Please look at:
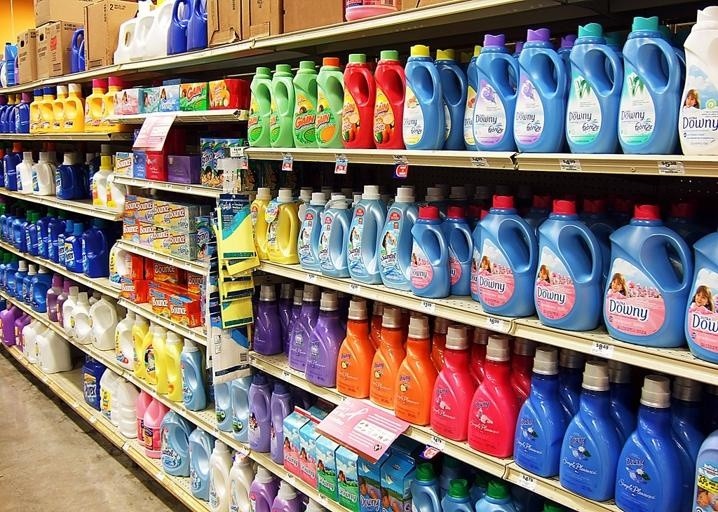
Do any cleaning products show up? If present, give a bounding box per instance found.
[1,1,718,512]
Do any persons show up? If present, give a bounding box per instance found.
[122,89,187,105]
[203,166,212,181]
[478,255,492,274]
[682,89,701,110]
[605,272,628,297]
[688,285,715,312]
[536,265,550,284]
[267,221,397,258]
[282,436,398,512]
[217,170,224,181]
[411,253,417,265]
